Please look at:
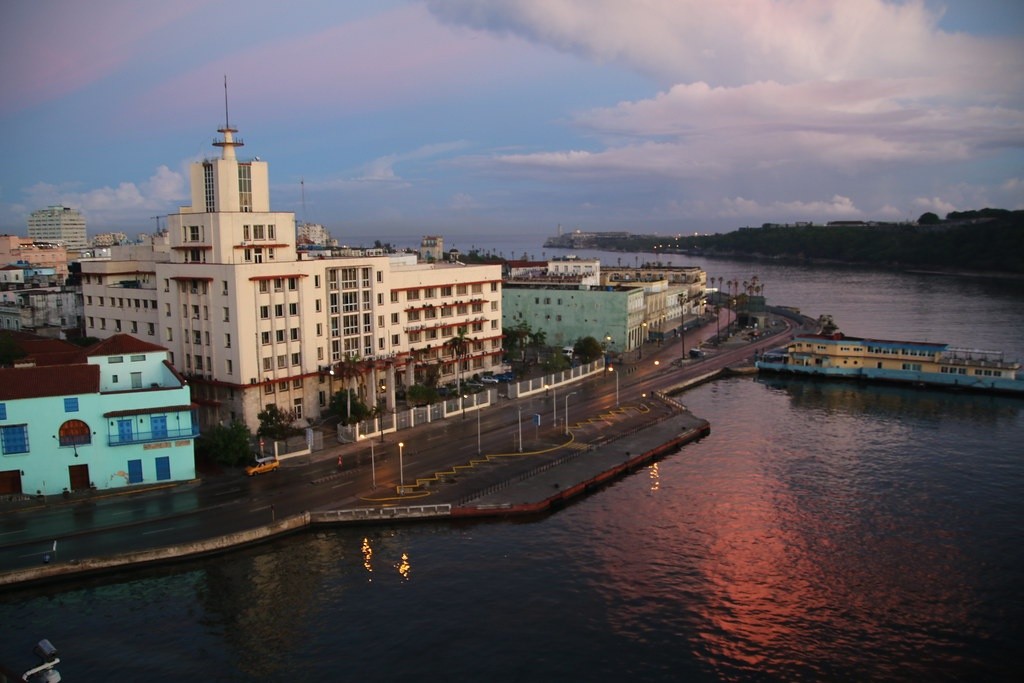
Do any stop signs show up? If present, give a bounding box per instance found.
[260,441,265,446]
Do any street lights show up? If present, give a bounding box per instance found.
[564,391,577,436]
[399,442,404,484]
[607,367,619,406]
[604,334,612,356]
[638,323,647,359]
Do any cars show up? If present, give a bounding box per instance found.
[244,455,279,477]
[562,346,580,360]
[438,373,514,398]
